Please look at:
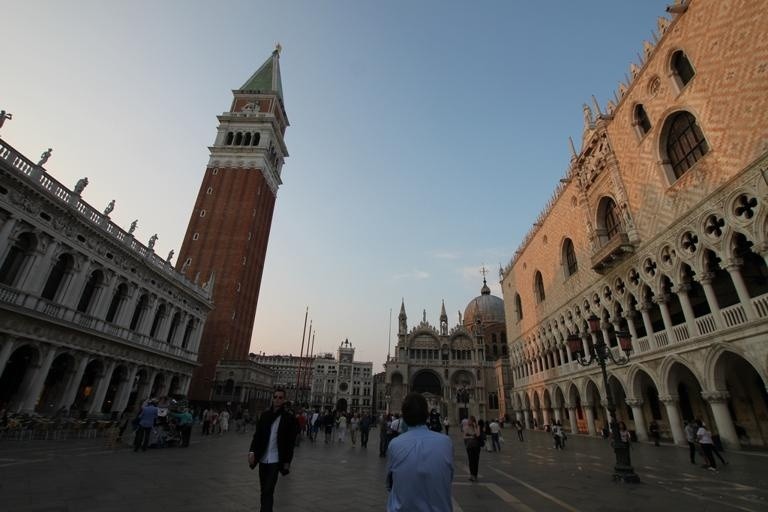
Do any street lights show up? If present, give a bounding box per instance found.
[457,382,475,419]
[565,313,642,483]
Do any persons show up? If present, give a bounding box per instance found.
[683,416,717,472]
[128,219,138,234]
[248,388,297,511]
[73,177,88,194]
[649,421,660,447]
[167,249,174,262]
[56,403,89,434]
[550,418,567,450]
[281,400,451,458]
[148,234,158,248]
[617,421,631,443]
[460,415,502,482]
[385,391,454,512]
[114,393,262,453]
[0,110,12,128]
[104,200,115,216]
[36,148,53,167]
[515,421,523,441]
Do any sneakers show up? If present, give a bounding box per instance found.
[469,475,476,481]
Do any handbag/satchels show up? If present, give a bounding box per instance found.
[473,425,486,446]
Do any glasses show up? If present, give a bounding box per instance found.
[273,395,284,399]
